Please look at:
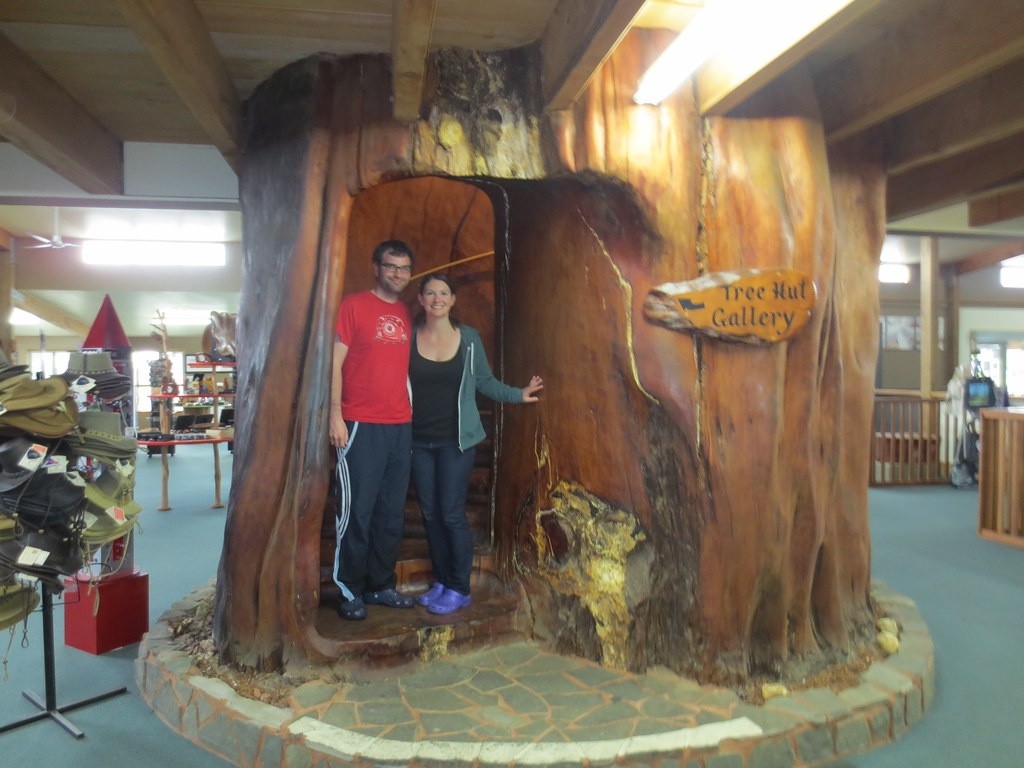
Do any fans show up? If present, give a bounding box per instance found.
[22,206,84,251]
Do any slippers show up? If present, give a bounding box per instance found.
[427,588,472,615]
[361,588,416,608]
[334,595,367,619]
[417,581,446,607]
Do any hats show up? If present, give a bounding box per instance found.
[0,349,141,630]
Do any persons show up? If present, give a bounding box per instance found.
[407,273,543,614]
[328,240,415,619]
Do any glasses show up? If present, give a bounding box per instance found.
[379,263,413,273]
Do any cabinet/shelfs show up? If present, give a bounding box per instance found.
[135,360,237,511]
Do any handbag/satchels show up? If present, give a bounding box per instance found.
[952,423,979,485]
[965,361,996,409]
[947,362,967,396]
